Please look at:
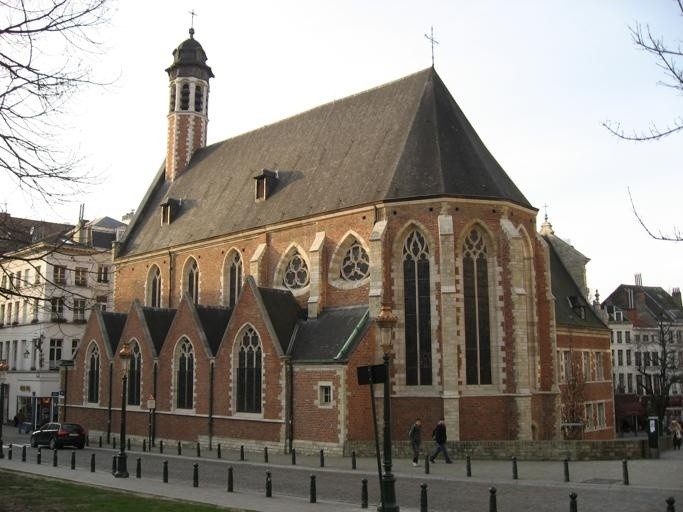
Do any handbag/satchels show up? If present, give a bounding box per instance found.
[676,431,683,440]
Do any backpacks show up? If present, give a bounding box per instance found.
[432,425,441,442]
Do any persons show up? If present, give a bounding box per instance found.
[15,408,23,435]
[408,418,422,466]
[429,417,453,463]
[670,419,681,450]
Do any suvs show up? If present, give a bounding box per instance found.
[30,422,85,449]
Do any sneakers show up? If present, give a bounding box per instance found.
[412,461,418,467]
[429,459,436,463]
[673,447,680,450]
[445,460,453,463]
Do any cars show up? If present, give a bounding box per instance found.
[662,406,683,432]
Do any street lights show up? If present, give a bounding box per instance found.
[113,342,132,477]
[375,300,399,511]
[0,370,8,460]
